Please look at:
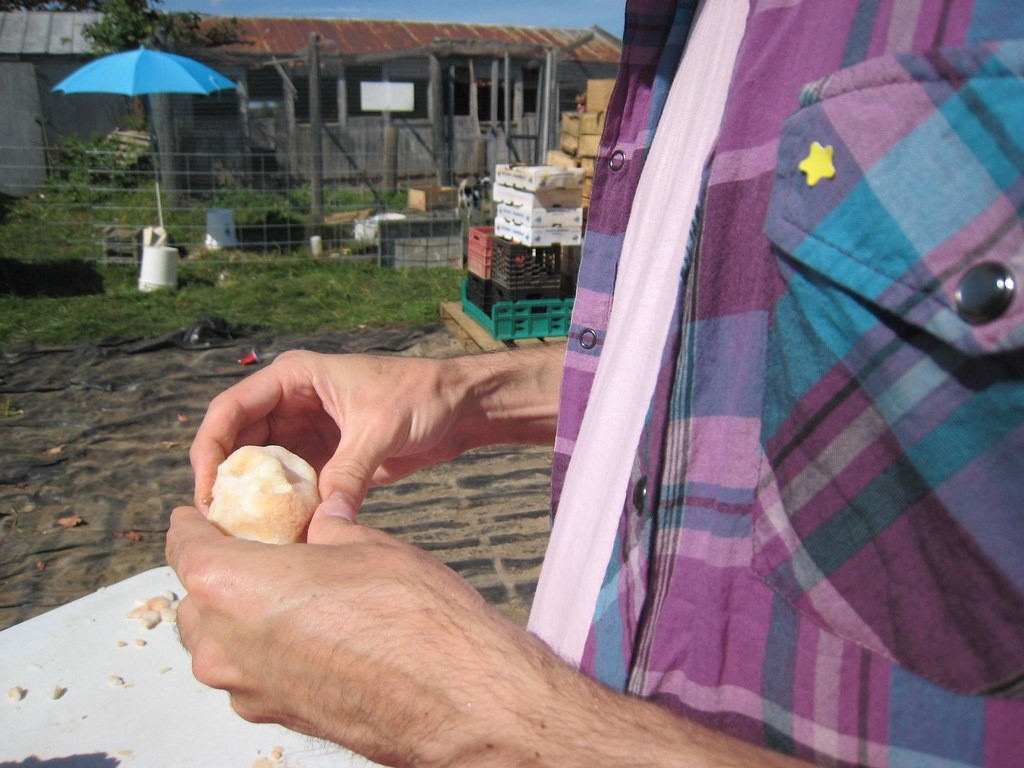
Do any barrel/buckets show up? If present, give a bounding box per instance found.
[138,246,178,293]
[205,209,237,248]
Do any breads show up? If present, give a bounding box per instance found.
[206,444,318,548]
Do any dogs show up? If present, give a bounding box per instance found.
[453,175,483,224]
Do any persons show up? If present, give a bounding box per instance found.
[165,1,1024,767]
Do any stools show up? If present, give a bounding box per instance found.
[102,223,144,267]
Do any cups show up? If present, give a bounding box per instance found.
[311,236,322,255]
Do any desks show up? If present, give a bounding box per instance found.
[0,566,385,768]
[379,208,463,269]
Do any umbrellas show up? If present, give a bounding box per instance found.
[50,44,239,229]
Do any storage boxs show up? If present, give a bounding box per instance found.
[325,210,380,239]
[460,77,618,340]
[408,184,460,212]
